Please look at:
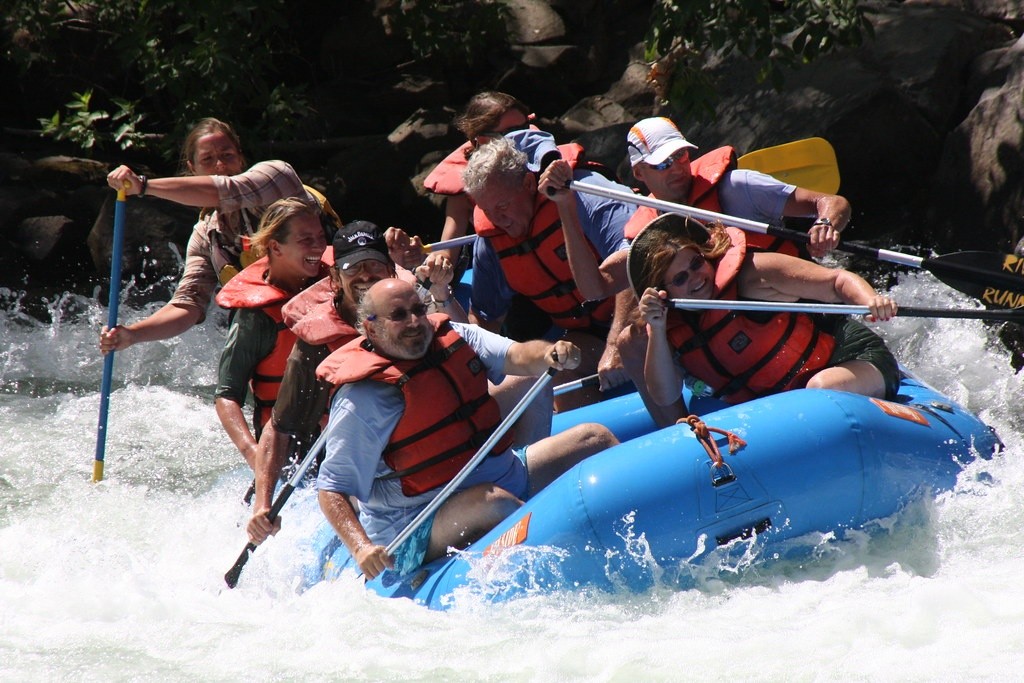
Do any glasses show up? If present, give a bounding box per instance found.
[643,149,690,170]
[376,304,428,322]
[335,261,386,276]
[666,254,706,289]
[479,123,530,140]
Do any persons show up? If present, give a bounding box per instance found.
[537,117,899,428]
[317,277,620,581]
[214,198,469,546]
[404,92,638,413]
[99,116,344,357]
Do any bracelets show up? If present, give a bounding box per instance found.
[431,285,454,308]
[138,175,147,198]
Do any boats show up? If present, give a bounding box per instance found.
[222,266,1005,609]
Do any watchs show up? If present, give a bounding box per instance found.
[811,217,832,227]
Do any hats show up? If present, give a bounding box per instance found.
[628,116,699,167]
[503,129,562,174]
[625,211,711,311]
[332,220,392,270]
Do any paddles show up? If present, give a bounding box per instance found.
[351,352,560,586]
[657,294,1024,320]
[415,136,840,252]
[93,189,127,483]
[547,175,1024,310]
[223,422,329,588]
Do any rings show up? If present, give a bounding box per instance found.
[573,357,579,361]
